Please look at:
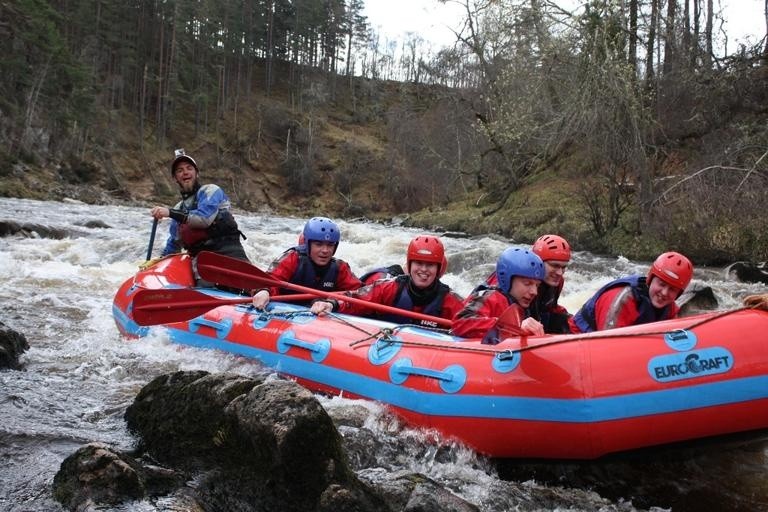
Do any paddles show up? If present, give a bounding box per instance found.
[197,251,454,327]
[133,289,358,326]
[482,303,534,345]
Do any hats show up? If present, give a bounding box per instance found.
[171,154,201,179]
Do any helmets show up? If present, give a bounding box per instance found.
[531,233,574,263]
[496,245,545,293]
[405,234,448,279]
[302,216,342,259]
[645,249,696,299]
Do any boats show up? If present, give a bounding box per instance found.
[110,255,767,460]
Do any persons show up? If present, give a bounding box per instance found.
[252,217,365,311]
[558,252,694,334]
[486,234,571,335]
[309,234,464,329]
[150,155,251,295]
[358,257,447,286]
[452,247,546,338]
